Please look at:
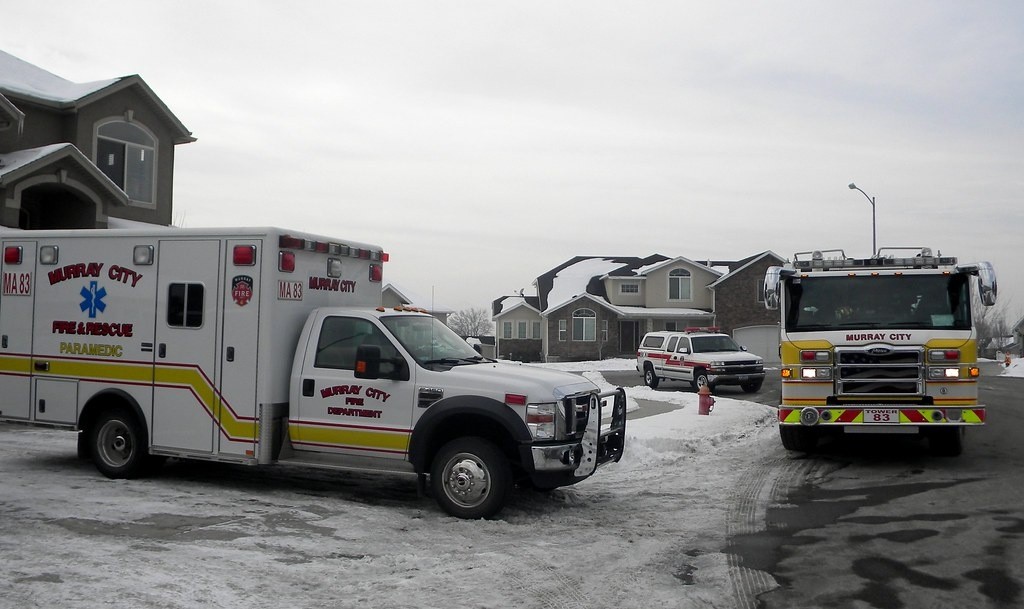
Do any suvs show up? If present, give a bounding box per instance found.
[635,326,766,395]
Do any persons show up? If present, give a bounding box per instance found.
[1004,350,1012,367]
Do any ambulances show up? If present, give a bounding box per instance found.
[1,226,629,522]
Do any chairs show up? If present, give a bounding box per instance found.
[324,329,361,365]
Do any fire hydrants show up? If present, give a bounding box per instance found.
[697,385,716,416]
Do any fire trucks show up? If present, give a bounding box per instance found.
[763,245,997,453]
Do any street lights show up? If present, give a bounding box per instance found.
[849,182,878,258]
